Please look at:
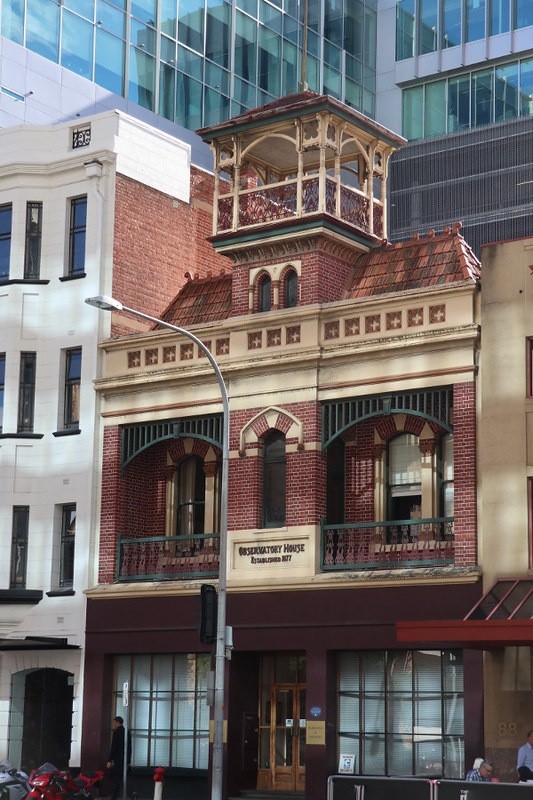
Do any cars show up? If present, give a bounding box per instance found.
[0,760,32,800]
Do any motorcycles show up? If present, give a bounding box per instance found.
[22,762,105,800]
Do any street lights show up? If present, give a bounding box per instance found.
[83,293,229,800]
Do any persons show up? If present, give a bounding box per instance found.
[465,761,493,782]
[517,730,533,784]
[107,717,132,800]
